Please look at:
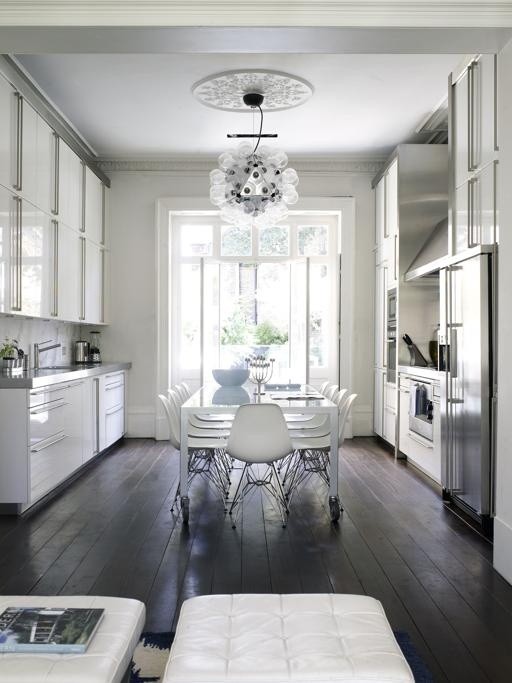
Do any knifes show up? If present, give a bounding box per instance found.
[402,334,412,344]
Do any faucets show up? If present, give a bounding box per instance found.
[33,340,61,369]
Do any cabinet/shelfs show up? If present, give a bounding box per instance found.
[447,52,498,254]
[370,154,399,452]
[2,72,109,325]
[1,370,124,518]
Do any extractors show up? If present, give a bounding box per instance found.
[405,218,448,284]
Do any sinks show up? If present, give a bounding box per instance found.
[35,366,76,378]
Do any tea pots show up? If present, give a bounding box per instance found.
[75,341,90,363]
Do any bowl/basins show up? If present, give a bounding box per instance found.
[213,369,250,386]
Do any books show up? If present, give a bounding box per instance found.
[0,607,106,653]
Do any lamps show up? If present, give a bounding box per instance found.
[192,68,321,229]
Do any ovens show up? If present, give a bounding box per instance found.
[398,373,439,488]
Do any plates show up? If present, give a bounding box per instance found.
[212,386,250,405]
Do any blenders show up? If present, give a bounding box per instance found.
[88,332,101,362]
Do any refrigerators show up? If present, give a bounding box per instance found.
[437,253,493,532]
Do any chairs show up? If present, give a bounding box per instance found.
[163,593,414,682]
[159,377,359,524]
[0,592,146,682]
